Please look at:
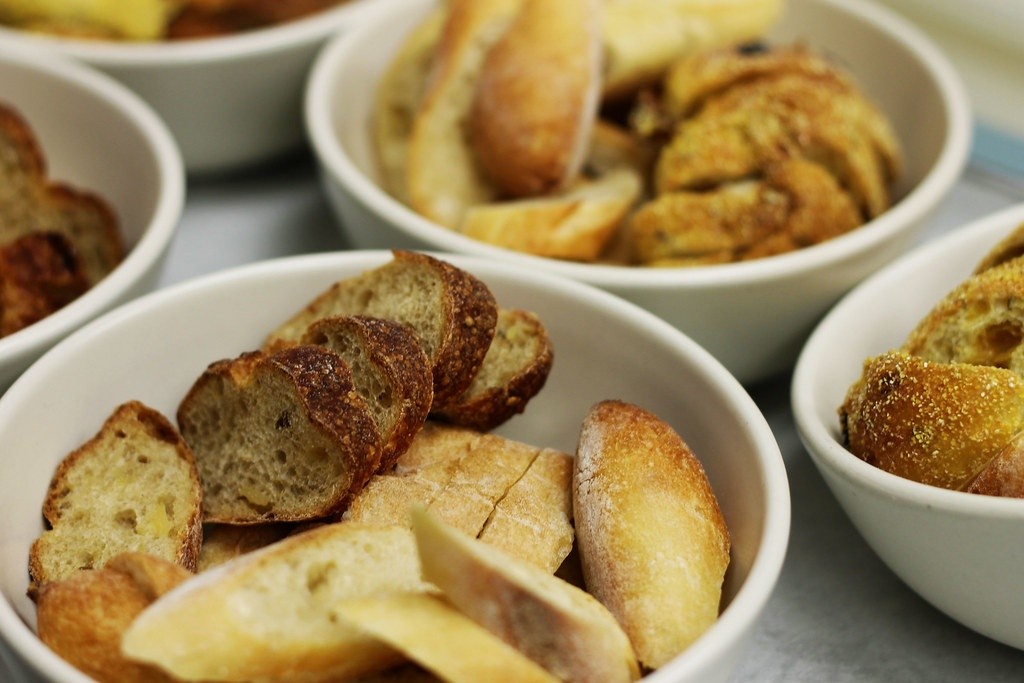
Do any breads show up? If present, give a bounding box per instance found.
[839,217,1023,502]
[366,0,904,266]
[23,252,733,683]
[0,0,351,340]
